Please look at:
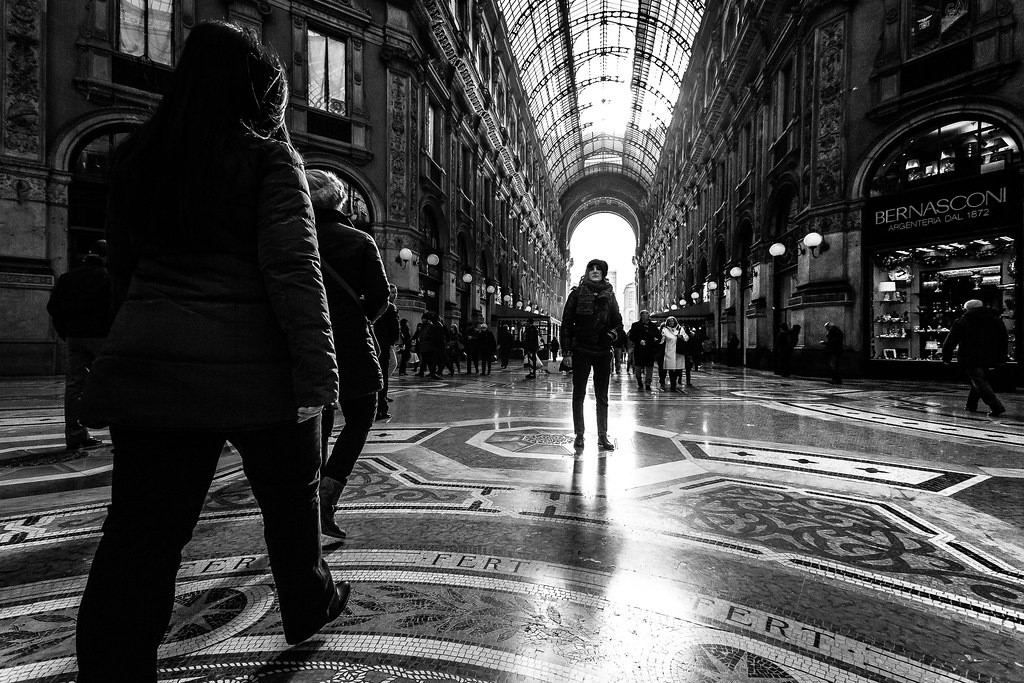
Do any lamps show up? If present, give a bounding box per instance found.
[395,246,523,311]
[670,228,832,311]
[878,281,895,301]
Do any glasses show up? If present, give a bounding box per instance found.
[480,326,487,330]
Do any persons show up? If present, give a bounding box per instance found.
[558,259,624,451]
[303,168,389,539]
[373,283,399,419]
[942,299,1009,417]
[610,310,801,393]
[48,239,109,450]
[76,20,351,682]
[819,321,844,385]
[396,310,560,380]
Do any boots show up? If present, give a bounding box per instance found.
[320,476,346,538]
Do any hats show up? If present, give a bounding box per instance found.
[964,299,982,310]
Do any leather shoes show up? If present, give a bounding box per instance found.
[326,580,351,622]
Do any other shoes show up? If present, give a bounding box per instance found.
[67,436,102,447]
[686,381,692,386]
[987,407,1006,416]
[598,435,614,449]
[575,437,583,446]
[671,382,676,387]
[525,374,536,379]
[646,384,651,390]
[963,404,978,412]
[638,382,643,387]
[678,377,681,383]
[398,368,491,380]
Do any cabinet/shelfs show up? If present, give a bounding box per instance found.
[999,287,1018,361]
[874,299,909,339]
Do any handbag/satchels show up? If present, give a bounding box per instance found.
[407,352,420,363]
[536,353,543,368]
[676,327,687,355]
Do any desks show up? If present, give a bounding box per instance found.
[914,329,951,360]
